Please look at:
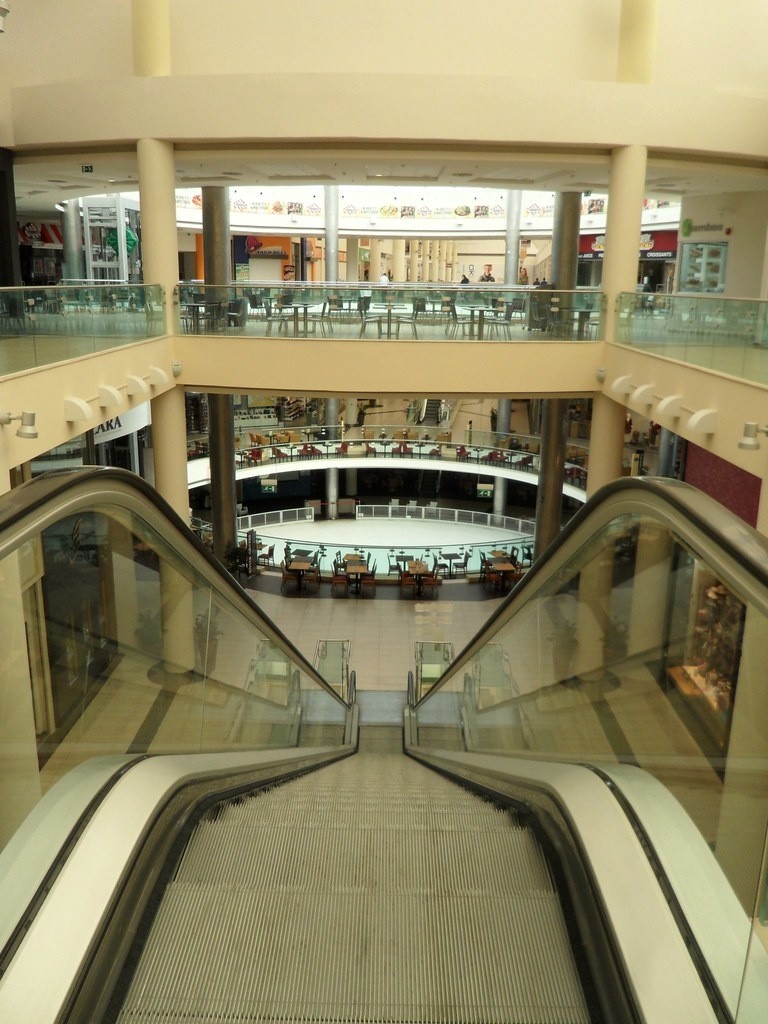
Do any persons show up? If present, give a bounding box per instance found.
[478,264,495,282]
[541,278,547,285]
[642,284,651,292]
[533,278,541,284]
[379,273,389,282]
[460,275,469,284]
[518,268,529,285]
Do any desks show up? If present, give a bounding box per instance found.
[459,305,489,338]
[441,552,462,579]
[265,434,277,445]
[396,555,414,571]
[373,307,407,339]
[292,549,313,557]
[562,309,600,339]
[510,442,523,451]
[256,544,267,550]
[337,299,359,324]
[557,306,580,334]
[408,561,428,596]
[292,556,314,574]
[263,297,276,315]
[289,562,311,593]
[415,445,425,459]
[286,447,296,461]
[473,448,483,464]
[421,434,431,441]
[380,442,390,458]
[303,432,314,441]
[236,453,247,468]
[425,300,443,325]
[506,453,517,469]
[180,303,218,334]
[344,554,359,560]
[498,301,520,326]
[379,434,388,439]
[347,560,366,587]
[347,566,367,594]
[464,308,498,341]
[281,305,314,338]
[322,444,332,458]
[487,551,510,558]
[289,302,321,333]
[487,557,516,592]
[375,303,404,308]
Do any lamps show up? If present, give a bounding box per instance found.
[738,421,768,450]
[0,409,38,439]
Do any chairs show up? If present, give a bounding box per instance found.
[132,517,641,599]
[389,499,399,513]
[185,416,588,488]
[408,500,417,513]
[427,501,438,515]
[1,283,635,341]
